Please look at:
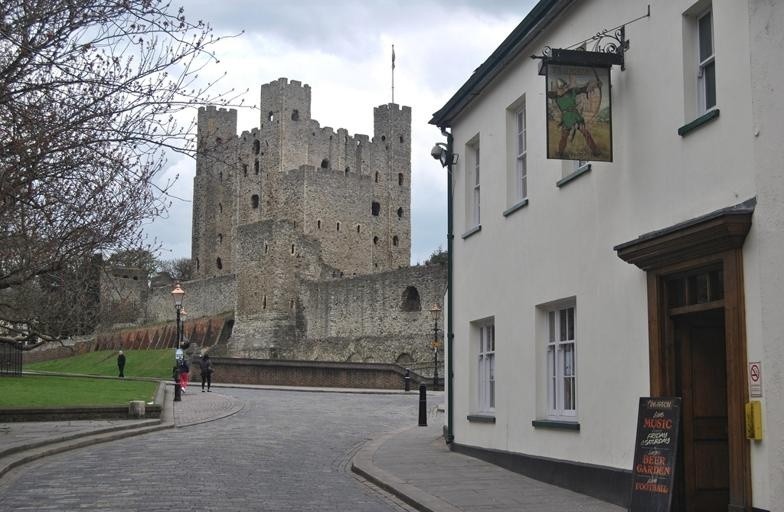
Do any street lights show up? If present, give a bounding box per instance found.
[429,303,442,390]
[179,307,187,351]
[171,283,186,401]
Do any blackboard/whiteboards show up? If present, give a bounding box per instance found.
[629,397,682,512]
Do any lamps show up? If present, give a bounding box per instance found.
[529,4,652,75]
[439,148,458,167]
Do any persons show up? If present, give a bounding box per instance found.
[117,350,126,377]
[179,360,189,393]
[199,353,215,392]
[546,77,603,159]
